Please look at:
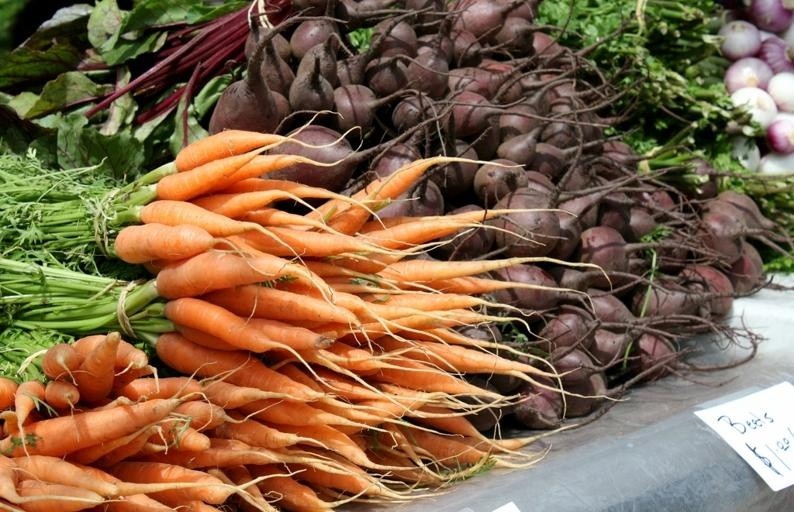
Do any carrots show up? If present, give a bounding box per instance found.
[0,111,630,512]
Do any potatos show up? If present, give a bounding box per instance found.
[207,0,794,430]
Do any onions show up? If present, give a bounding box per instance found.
[717,0,794,175]
